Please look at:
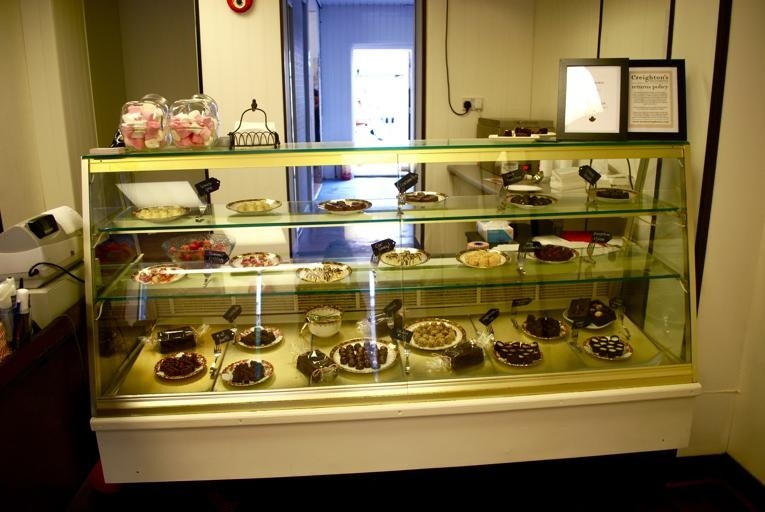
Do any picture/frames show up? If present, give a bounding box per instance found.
[630,59,687,140]
[558,57,629,140]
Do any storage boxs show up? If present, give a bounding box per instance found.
[477,118,555,176]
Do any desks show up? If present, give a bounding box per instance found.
[448,165,552,221]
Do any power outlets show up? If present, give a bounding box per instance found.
[459,95,475,111]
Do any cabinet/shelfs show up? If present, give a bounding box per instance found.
[81,142,701,486]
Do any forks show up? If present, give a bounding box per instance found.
[210,345,222,376]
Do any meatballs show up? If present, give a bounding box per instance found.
[339,340,387,370]
[411,321,456,347]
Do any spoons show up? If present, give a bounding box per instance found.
[404,345,411,375]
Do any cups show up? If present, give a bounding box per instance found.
[301,309,343,338]
[539,159,608,177]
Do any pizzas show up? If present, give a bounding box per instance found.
[236,201,271,212]
[139,267,177,283]
[137,207,188,218]
[302,264,349,283]
[385,249,424,267]
[238,253,271,268]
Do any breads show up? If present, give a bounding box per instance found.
[405,192,439,201]
[535,245,574,260]
[232,361,263,384]
[567,297,617,331]
[510,194,552,206]
[297,350,339,384]
[595,189,629,199]
[242,326,275,346]
[527,314,561,336]
[462,249,501,266]
[326,200,366,210]
[496,127,549,136]
[160,354,203,377]
[157,325,196,352]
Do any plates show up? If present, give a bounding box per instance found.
[586,188,639,203]
[527,244,579,264]
[522,320,568,340]
[131,263,188,286]
[492,343,543,367]
[132,206,192,223]
[488,134,539,145]
[222,359,273,386]
[315,198,372,215]
[331,337,398,374]
[562,308,616,329]
[456,248,511,269]
[379,246,432,268]
[226,198,282,215]
[536,131,556,142]
[584,336,634,360]
[396,191,448,208]
[228,251,282,268]
[161,233,236,270]
[154,352,206,380]
[503,184,544,191]
[235,326,283,348]
[296,261,352,284]
[505,193,558,210]
[406,321,463,350]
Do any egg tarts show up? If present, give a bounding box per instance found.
[496,341,541,365]
[590,334,624,358]
